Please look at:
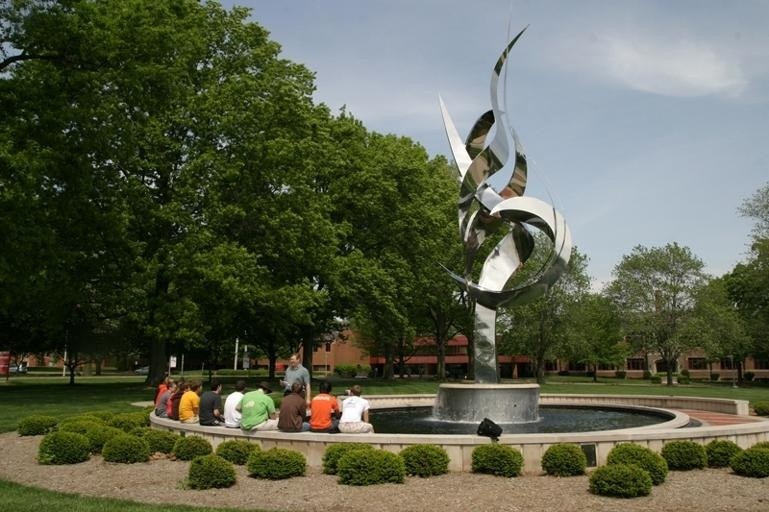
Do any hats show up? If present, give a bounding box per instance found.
[256,380,272,394]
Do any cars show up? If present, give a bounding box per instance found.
[135,365,150,375]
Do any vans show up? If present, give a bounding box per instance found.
[8,363,28,376]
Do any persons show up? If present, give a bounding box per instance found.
[154,374,375,434]
[282,353,312,407]
